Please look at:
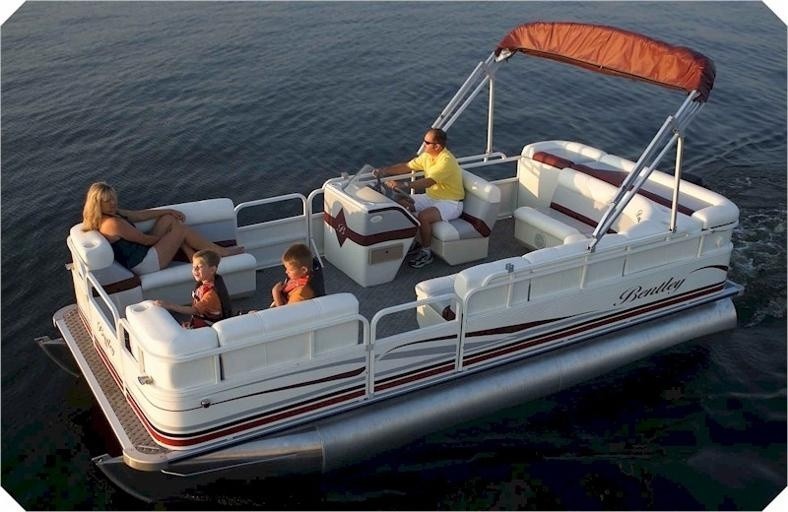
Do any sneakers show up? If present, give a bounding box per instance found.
[408,244,434,269]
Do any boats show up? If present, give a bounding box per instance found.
[34,20,744,506]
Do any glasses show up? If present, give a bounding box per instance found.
[424,140,440,144]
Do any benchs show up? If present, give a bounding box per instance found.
[413,165,661,329]
[70,199,262,312]
[208,290,369,381]
[420,165,504,272]
[121,299,223,390]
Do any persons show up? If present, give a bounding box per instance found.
[373,129,465,268]
[83,182,245,276]
[235,244,324,317]
[153,249,232,330]
